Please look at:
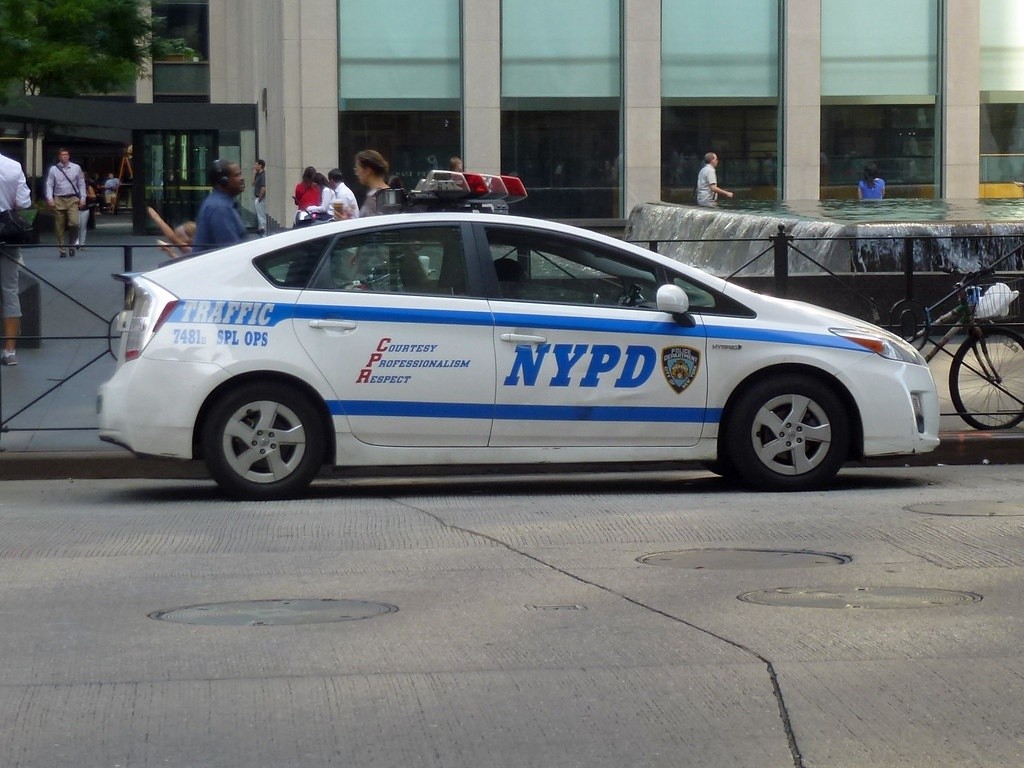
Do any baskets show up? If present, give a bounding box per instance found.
[964,277,1022,321]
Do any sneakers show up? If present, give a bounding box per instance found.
[0,354,18,365]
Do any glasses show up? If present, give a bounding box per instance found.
[353,167,360,172]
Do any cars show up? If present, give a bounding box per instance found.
[91,171,942,502]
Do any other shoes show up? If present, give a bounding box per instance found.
[69,248,75,256]
[61,253,66,257]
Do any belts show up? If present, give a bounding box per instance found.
[58,194,77,197]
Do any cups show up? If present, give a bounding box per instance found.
[333,202,344,216]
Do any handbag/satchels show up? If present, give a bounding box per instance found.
[86,185,97,198]
[79,197,88,210]
[0,208,40,244]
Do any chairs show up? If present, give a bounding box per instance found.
[439,252,468,296]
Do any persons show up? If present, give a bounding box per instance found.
[295,151,400,216]
[493,257,531,300]
[193,159,249,250]
[694,152,733,200]
[449,157,463,171]
[46,148,87,258]
[106,173,121,213]
[147,207,195,259]
[858,163,884,200]
[76,172,96,251]
[252,160,265,236]
[0,151,32,366]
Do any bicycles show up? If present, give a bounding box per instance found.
[901,263,1024,429]
